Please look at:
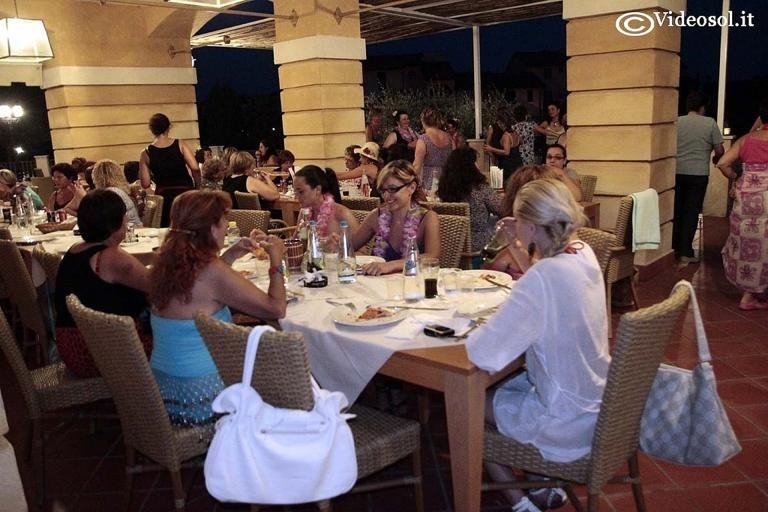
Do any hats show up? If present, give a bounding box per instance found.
[353,141,385,164]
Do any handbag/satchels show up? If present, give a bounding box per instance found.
[635,278,744,471]
[203,325,359,508]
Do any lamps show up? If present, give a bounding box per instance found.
[0,0,54,63]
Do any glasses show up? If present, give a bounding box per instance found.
[546,154,566,161]
[377,177,417,194]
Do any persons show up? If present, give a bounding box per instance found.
[462,178,612,512]
[716,99,768,310]
[53,189,156,376]
[146,189,288,428]
[671,92,726,262]
[0,102,583,278]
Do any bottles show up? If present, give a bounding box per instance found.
[275,177,295,198]
[430,170,439,201]
[0,193,67,235]
[303,221,327,282]
[226,221,239,244]
[336,220,357,284]
[402,230,421,303]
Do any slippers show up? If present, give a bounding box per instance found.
[738,297,768,310]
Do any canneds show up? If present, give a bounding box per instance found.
[279,179,287,193]
[47,211,56,222]
[59,210,67,221]
[288,180,293,185]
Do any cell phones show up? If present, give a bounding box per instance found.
[424,323,456,338]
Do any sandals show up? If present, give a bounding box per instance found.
[507,474,570,512]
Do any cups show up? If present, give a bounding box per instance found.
[339,171,373,198]
[421,254,477,308]
[480,223,517,264]
[281,239,303,273]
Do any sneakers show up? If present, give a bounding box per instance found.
[679,254,701,264]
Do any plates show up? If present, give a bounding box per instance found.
[332,304,407,327]
[458,270,512,289]
[227,250,299,302]
[53,234,84,253]
[12,236,55,243]
[354,255,387,272]
[122,228,168,257]
